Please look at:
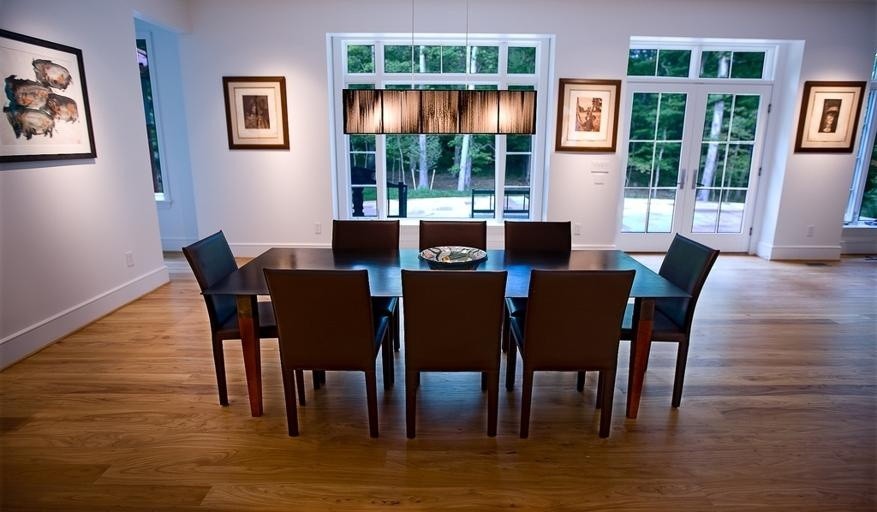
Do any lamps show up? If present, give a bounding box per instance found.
[343,0,537,134]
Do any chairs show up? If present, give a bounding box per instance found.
[333,221,399,386]
[263,269,391,438]
[182,231,320,406]
[402,270,507,437]
[420,221,486,250]
[502,222,571,385]
[508,270,636,438]
[596,234,720,410]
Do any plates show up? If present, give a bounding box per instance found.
[419,246,488,265]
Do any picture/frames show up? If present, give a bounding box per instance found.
[794,81,867,153]
[222,76,289,149]
[0,29,97,162]
[555,79,621,152]
